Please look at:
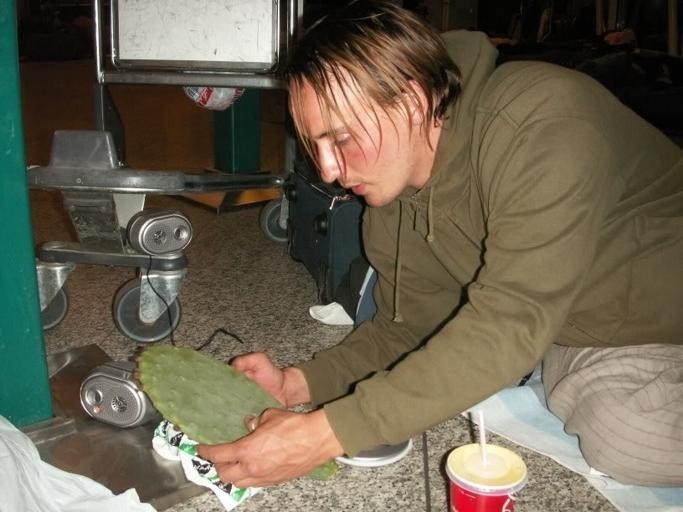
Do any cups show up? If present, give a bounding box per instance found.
[445,442,529,512]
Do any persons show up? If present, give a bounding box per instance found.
[194,0,683,488]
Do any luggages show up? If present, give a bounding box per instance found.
[283,164,362,304]
